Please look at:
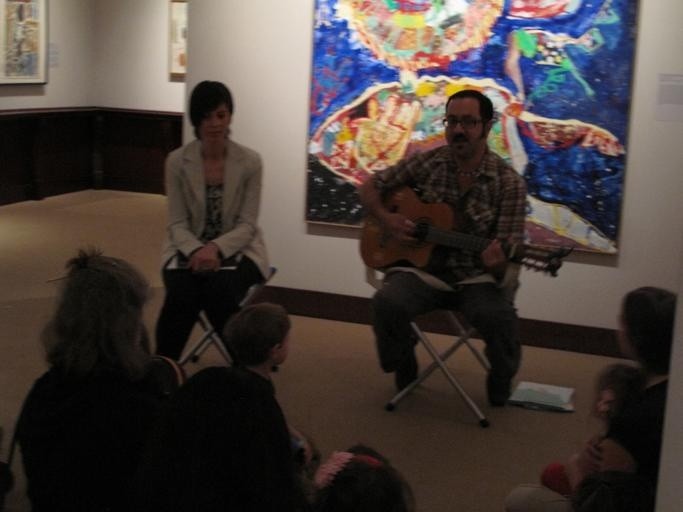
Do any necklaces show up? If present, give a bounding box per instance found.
[454,152,479,178]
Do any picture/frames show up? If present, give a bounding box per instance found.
[167,0,188,76]
[0,0,48,84]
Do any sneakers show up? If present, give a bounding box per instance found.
[392,336,419,391]
[485,366,513,407]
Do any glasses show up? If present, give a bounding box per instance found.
[442,117,487,129]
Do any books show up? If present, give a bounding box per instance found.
[175,249,188,266]
[508,377,575,411]
[164,253,244,272]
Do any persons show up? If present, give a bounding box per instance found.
[125,301,314,511]
[357,90,530,407]
[154,80,282,372]
[500,285,676,512]
[14,248,191,508]
[314,442,413,511]
[539,360,644,498]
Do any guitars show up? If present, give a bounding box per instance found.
[360,186,563,277]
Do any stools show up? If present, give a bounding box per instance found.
[387,312,493,427]
[178,264,278,366]
